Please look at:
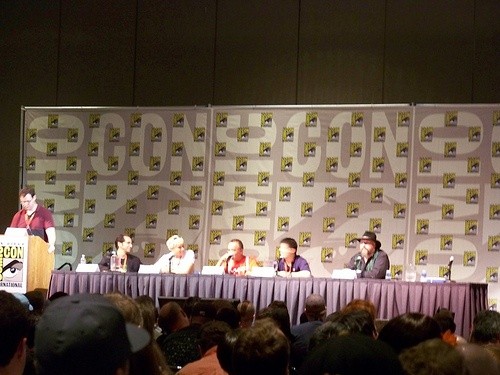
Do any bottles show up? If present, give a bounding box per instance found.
[120,254,128,273]
[193,252,202,276]
[404,262,417,282]
[80,254,86,264]
[110,250,120,272]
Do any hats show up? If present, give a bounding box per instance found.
[12,290,71,314]
[434,307,455,323]
[34,294,150,375]
[302,333,408,375]
[349,231,382,251]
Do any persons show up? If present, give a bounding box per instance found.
[152,235,195,274]
[11,188,56,252]
[35,294,151,375]
[17,290,69,375]
[98,234,142,272]
[217,239,258,274]
[348,232,389,279]
[274,238,311,277]
[0,290,28,375]
[109,291,500,375]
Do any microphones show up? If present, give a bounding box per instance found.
[448,256,454,266]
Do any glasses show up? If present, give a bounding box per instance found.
[20,199,32,204]
[179,245,184,248]
[127,242,132,245]
[227,249,236,252]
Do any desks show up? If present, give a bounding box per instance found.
[48,271,489,345]
